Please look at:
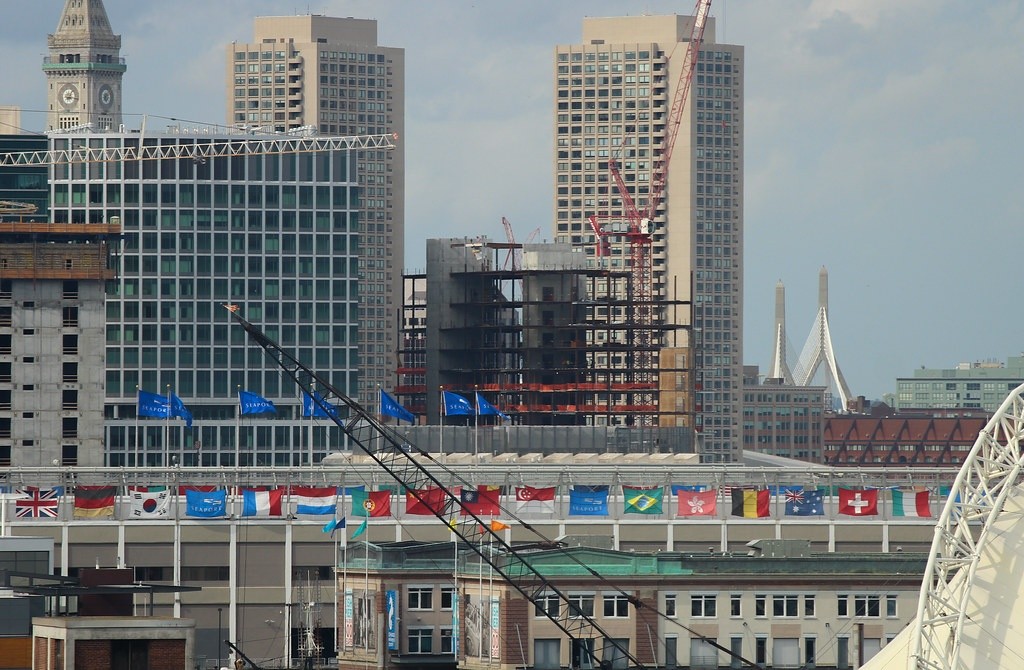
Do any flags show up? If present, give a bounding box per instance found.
[380,388,414,424]
[313,392,344,427]
[477,391,510,421]
[238,389,278,415]
[442,390,476,416]
[137,389,169,418]
[299,391,312,417]
[168,390,193,430]
[14,485,986,539]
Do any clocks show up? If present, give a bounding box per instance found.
[99,83,114,108]
[57,83,79,108]
